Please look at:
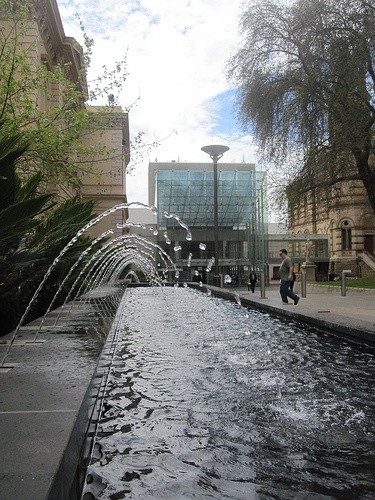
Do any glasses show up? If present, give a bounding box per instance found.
[279,252,283,256]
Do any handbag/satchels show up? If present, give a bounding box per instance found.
[288,272,296,281]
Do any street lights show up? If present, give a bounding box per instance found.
[200,144,230,288]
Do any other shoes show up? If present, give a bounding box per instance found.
[294,295,299,305]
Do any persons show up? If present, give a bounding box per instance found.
[249,269,257,293]
[278,249,300,305]
